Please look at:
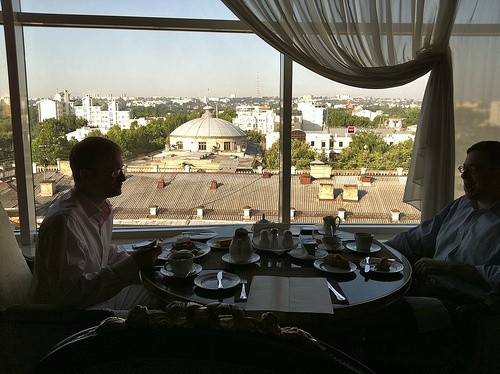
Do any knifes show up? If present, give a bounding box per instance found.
[327,281,346,301]
[364,257,370,273]
[216,271,223,289]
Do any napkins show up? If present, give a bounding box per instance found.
[245,275,334,315]
[402,294,453,335]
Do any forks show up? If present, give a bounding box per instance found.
[239,275,248,300]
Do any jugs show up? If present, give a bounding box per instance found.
[229,227,253,263]
[323,215,340,234]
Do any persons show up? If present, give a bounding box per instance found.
[30,138,166,312]
[383,142,500,309]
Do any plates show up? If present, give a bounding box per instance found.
[346,242,381,253]
[159,263,203,277]
[313,259,358,273]
[154,241,211,260]
[206,236,233,249]
[221,252,261,264]
[182,230,217,240]
[317,243,345,251]
[251,237,299,252]
[360,257,404,273]
[289,248,328,259]
[194,272,240,289]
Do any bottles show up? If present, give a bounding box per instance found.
[319,229,341,235]
[259,228,294,249]
[252,214,273,239]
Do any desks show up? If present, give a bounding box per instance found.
[140,231,413,356]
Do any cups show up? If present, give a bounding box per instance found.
[312,224,333,244]
[354,232,374,251]
[164,252,195,275]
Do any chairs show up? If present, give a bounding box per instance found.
[0,202,117,374]
[28,301,379,374]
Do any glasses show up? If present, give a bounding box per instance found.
[97,164,126,177]
[459,164,497,174]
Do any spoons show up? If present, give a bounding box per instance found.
[184,266,197,278]
